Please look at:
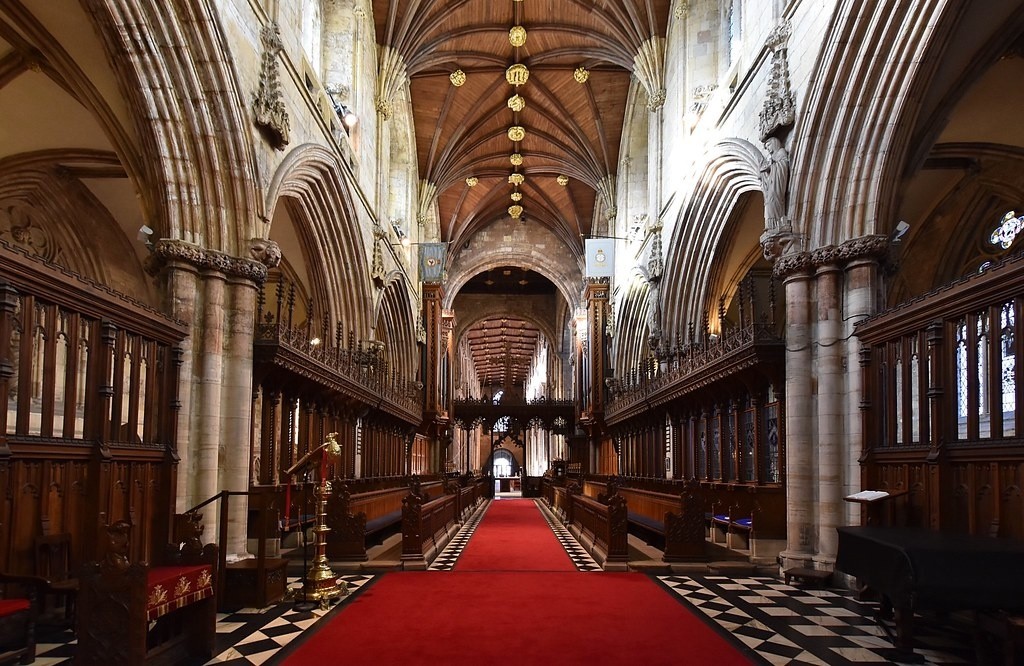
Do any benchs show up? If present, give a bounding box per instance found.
[324,473,484,570]
[553,473,787,562]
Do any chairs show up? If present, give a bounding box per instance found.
[34,533,78,620]
[0,572,51,666]
[77,439,219,666]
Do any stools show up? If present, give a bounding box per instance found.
[976,608,1024,666]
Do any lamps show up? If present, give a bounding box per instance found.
[334,103,357,137]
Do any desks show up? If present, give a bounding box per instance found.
[835,527,1024,666]
[224,558,289,608]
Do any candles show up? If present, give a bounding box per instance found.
[321,449,326,487]
